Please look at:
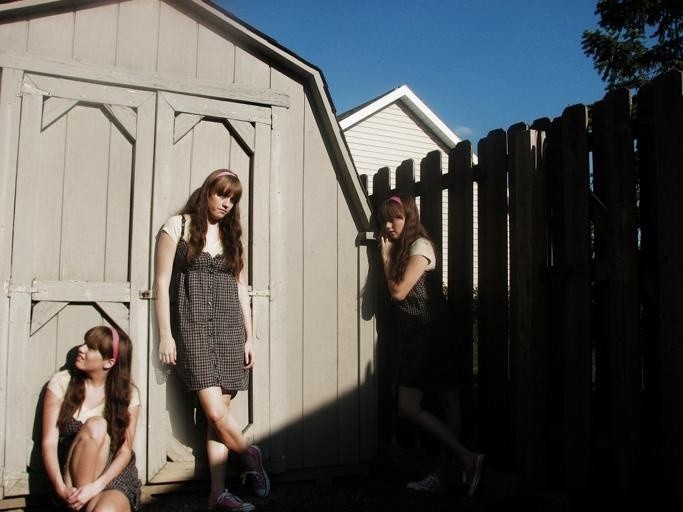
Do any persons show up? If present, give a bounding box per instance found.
[153,167,272,511]
[374,188,487,498]
[40,325,143,511]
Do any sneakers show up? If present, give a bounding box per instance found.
[461,451,487,500]
[406,470,445,493]
[206,488,256,512]
[240,444,271,498]
